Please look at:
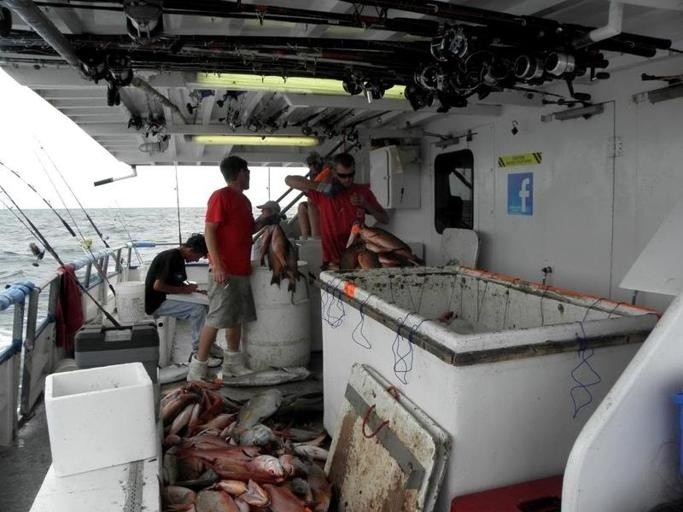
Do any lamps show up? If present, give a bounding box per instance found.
[75,0,683,146]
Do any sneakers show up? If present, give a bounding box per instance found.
[188,342,224,368]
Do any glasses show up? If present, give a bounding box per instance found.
[334,170,355,179]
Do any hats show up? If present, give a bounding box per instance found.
[256,200,281,214]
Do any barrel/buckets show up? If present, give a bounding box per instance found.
[240,261,313,371]
[115,280,145,322]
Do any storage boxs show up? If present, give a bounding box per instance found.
[43,362,157,476]
[74,320,160,385]
[28,417,164,510]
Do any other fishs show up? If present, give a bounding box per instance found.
[258,223,297,292]
[340,223,421,268]
[161,383,337,512]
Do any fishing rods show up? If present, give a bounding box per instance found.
[2,0,673,141]
[0,137,144,330]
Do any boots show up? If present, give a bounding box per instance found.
[187,354,209,383]
[221,349,254,379]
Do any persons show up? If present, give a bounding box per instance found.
[285,151,390,265]
[143,233,224,368]
[185,154,258,386]
[255,200,281,228]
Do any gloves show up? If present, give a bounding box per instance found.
[317,182,334,195]
